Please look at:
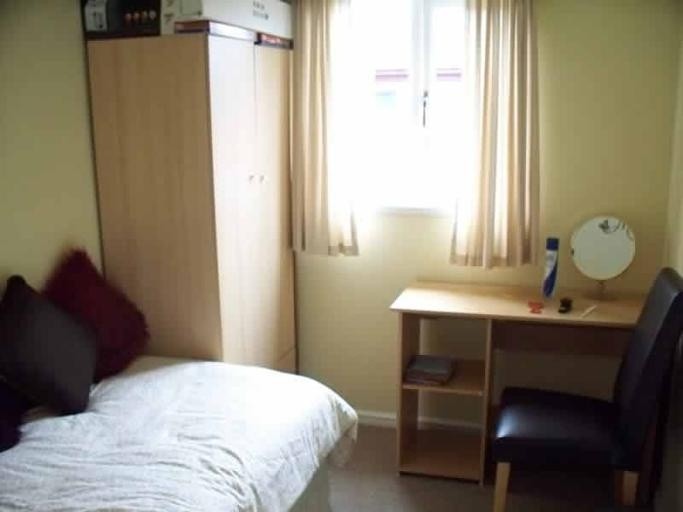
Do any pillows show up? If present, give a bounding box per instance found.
[2,240,147,447]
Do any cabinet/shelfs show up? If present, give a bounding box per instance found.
[81,32,301,376]
[386,313,493,491]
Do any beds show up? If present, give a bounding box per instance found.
[2,347,356,511]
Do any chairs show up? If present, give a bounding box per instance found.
[489,265,683,510]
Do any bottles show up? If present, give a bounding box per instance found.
[542,237,560,302]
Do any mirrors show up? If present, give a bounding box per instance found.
[569,213,640,302]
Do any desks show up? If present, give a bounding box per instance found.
[389,277,657,332]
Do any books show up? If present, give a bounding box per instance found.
[406,353,455,387]
